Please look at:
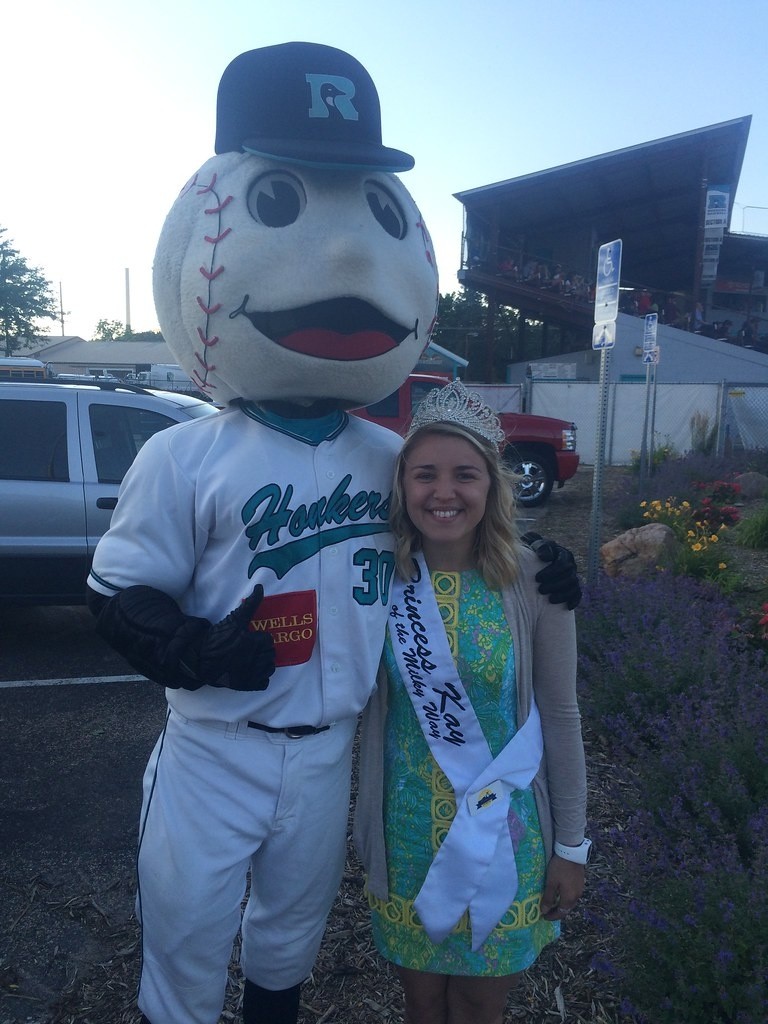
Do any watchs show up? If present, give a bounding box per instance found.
[554,838,593,865]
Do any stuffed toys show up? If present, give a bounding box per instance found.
[84,40,581,1024]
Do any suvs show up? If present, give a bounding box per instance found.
[345,371,580,509]
[0,355,235,613]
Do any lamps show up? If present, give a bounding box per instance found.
[636,345,642,356]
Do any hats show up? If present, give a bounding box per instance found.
[213,40,416,174]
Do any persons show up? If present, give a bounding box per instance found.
[356,378,590,1023]
[502,258,760,347]
